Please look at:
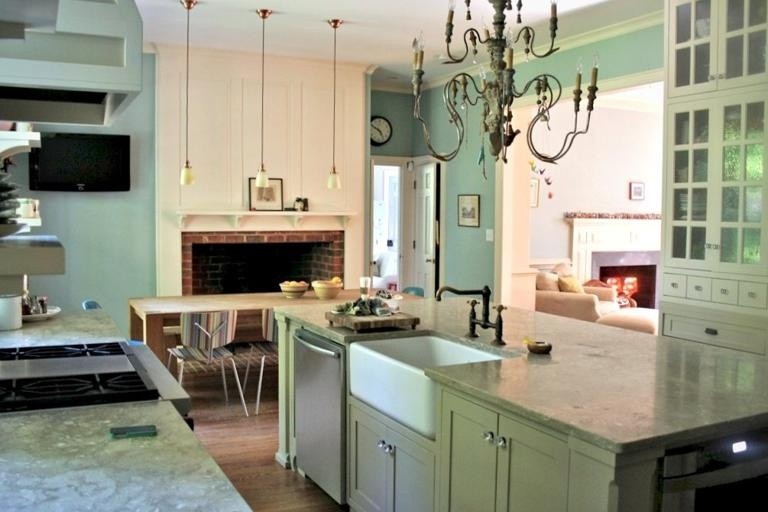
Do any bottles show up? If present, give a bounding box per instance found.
[369,261,379,277]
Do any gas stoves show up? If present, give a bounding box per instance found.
[0,340,190,412]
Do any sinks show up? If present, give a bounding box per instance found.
[348,334,505,439]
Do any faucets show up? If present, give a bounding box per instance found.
[435,285,491,323]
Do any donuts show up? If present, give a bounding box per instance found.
[528,339,552,354]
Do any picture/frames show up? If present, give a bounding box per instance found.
[247,177,284,212]
[628,181,645,201]
[529,178,539,209]
[456,194,480,228]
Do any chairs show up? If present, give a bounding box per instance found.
[161,309,249,418]
[241,308,277,415]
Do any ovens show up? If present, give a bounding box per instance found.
[291,328,346,508]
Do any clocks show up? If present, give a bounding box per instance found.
[369,115,392,145]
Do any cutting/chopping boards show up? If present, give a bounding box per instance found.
[325,310,422,333]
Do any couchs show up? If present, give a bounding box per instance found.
[536,270,619,322]
[596,308,659,334]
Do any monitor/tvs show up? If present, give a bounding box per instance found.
[28,132,130,192]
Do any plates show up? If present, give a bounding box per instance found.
[674,165,704,183]
[22,306,62,322]
[677,191,706,220]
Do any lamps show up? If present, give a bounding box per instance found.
[178,0,199,188]
[251,9,274,188]
[327,18,343,191]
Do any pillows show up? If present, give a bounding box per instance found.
[558,274,584,294]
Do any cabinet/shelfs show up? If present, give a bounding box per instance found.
[667,0,767,96]
[665,87,768,278]
[662,272,767,311]
[438,390,567,511]
[346,404,434,511]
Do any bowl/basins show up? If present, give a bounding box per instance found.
[280,284,309,299]
[311,279,343,300]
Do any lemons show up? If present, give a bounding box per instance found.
[281,280,307,287]
[332,276,341,283]
